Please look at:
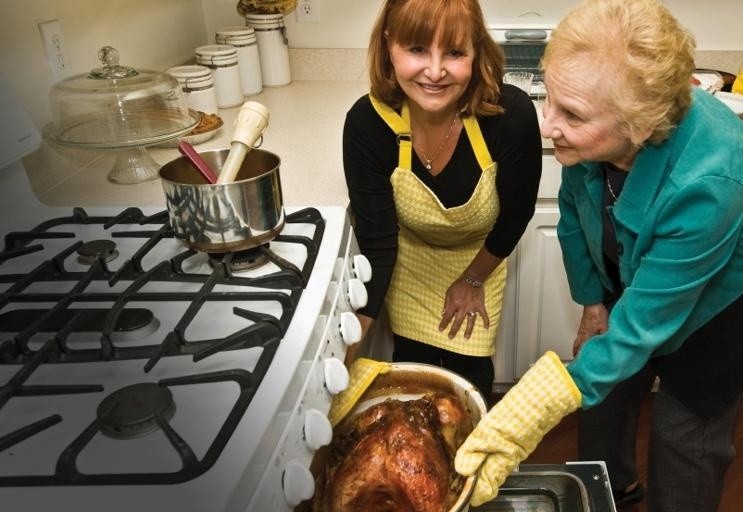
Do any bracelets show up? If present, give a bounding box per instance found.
[461,274,482,288]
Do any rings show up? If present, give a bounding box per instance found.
[466,312,476,317]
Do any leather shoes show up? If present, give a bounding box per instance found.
[613,480,645,509]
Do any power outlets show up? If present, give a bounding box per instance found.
[39,19,71,78]
[295,0,321,23]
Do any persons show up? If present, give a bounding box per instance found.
[343,0,541,402]
[454,0,743,511]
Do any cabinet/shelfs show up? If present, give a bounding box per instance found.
[492,196,658,386]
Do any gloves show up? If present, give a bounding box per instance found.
[453,349,583,508]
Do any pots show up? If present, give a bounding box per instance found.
[157,148,287,255]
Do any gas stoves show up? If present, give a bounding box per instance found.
[0,207,367,512]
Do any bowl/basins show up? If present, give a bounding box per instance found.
[342,362,489,512]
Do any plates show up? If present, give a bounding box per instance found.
[154,113,225,145]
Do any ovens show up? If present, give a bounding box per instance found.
[228,208,625,512]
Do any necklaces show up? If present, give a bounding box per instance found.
[411,107,461,171]
[605,176,618,201]
